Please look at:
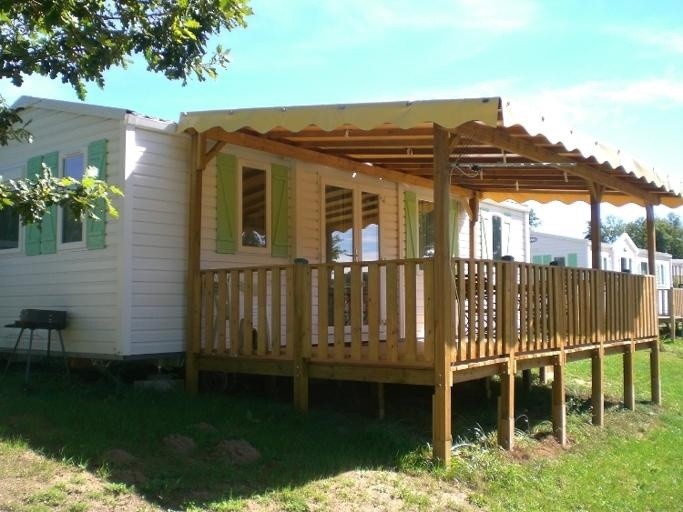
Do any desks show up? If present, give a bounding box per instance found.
[0,308,72,393]
